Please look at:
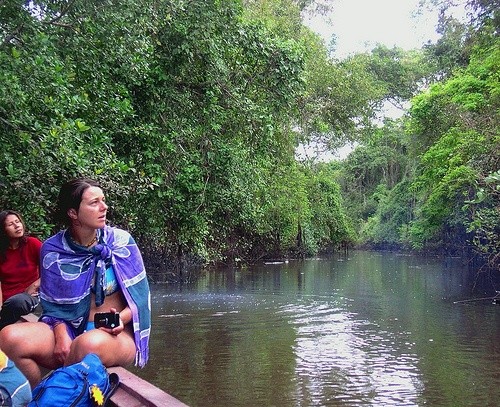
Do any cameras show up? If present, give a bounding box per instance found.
[94,312,119,329]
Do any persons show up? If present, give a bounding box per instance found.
[0,210,44,329]
[0,179,150,395]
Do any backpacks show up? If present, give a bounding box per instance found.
[28,353,121,407]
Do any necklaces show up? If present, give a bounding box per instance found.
[69,229,97,247]
